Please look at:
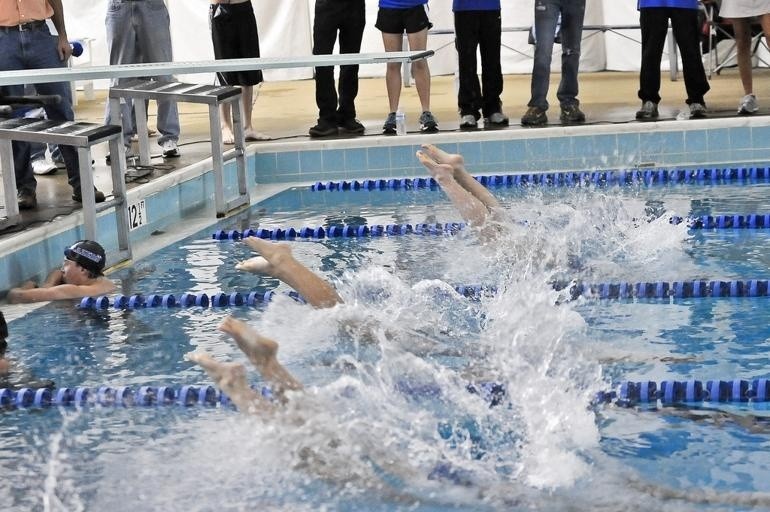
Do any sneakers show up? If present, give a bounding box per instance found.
[30,160,58,176]
[105,145,134,159]
[521,104,547,127]
[687,104,708,119]
[560,105,586,124]
[419,110,439,134]
[309,124,338,138]
[336,119,365,135]
[483,112,509,126]
[16,190,37,209]
[636,101,659,120]
[160,138,181,159]
[71,186,105,203]
[459,114,478,128]
[737,94,759,114]
[383,112,396,135]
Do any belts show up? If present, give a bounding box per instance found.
[0,19,46,32]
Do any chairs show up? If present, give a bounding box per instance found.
[701,0,770,79]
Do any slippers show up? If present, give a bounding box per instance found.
[245,132,271,142]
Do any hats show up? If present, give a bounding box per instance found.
[63,239,106,273]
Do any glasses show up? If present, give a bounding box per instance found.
[62,245,73,259]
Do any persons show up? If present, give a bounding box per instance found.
[183,317,770,509]
[233,235,701,366]
[22,141,95,176]
[450,0,511,130]
[634,0,711,121]
[307,1,368,136]
[208,1,274,143]
[7,241,152,340]
[1,311,57,390]
[102,0,182,161]
[1,1,105,209]
[521,0,587,125]
[131,97,155,141]
[716,0,770,116]
[374,0,439,131]
[415,142,508,249]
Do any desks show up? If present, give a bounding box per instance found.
[64,37,99,109]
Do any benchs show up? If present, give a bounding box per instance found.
[395,23,682,88]
